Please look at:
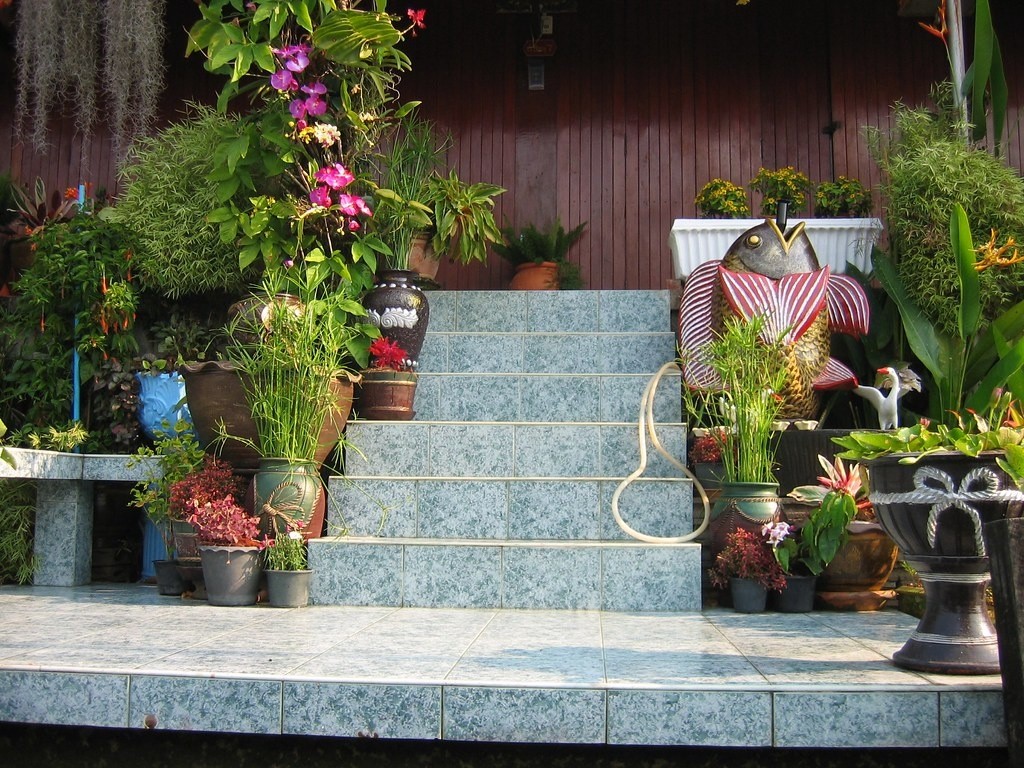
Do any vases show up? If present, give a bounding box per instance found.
[175,533,199,556]
[815,521,899,612]
[179,361,362,468]
[266,569,314,607]
[196,545,263,606]
[730,578,767,613]
[353,366,418,421]
[770,575,818,613]
[136,372,209,451]
[666,217,884,280]
[227,293,307,353]
[694,462,736,490]
[859,449,1019,675]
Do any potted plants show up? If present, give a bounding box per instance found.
[223,261,388,551]
[362,101,461,371]
[672,301,794,565]
[124,416,206,596]
[490,213,589,291]
[375,160,509,281]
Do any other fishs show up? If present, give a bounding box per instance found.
[679,216,870,428]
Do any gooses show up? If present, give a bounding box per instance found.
[850,366,917,429]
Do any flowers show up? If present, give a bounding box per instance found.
[185,493,276,550]
[167,454,246,532]
[182,0,429,268]
[688,428,738,466]
[23,181,143,365]
[708,527,788,594]
[786,453,879,575]
[828,228,1024,466]
[259,520,311,571]
[760,520,799,574]
[693,165,874,219]
[367,336,412,371]
